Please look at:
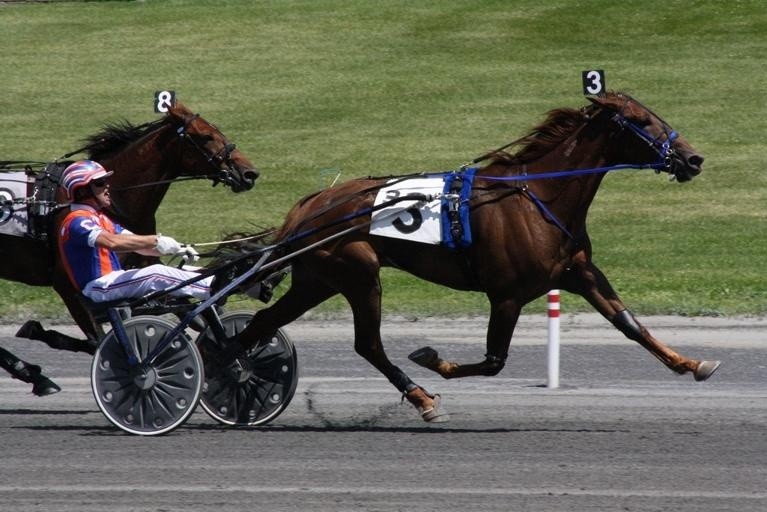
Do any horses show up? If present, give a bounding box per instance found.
[193,88,723,424]
[0,97,261,398]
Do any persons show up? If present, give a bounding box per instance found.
[58,159,287,304]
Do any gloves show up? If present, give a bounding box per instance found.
[152,232,200,265]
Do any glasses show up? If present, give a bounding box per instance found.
[93,180,105,187]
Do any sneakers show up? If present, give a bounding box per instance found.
[259,272,288,304]
[210,266,235,306]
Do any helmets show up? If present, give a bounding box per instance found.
[60,160,115,203]
